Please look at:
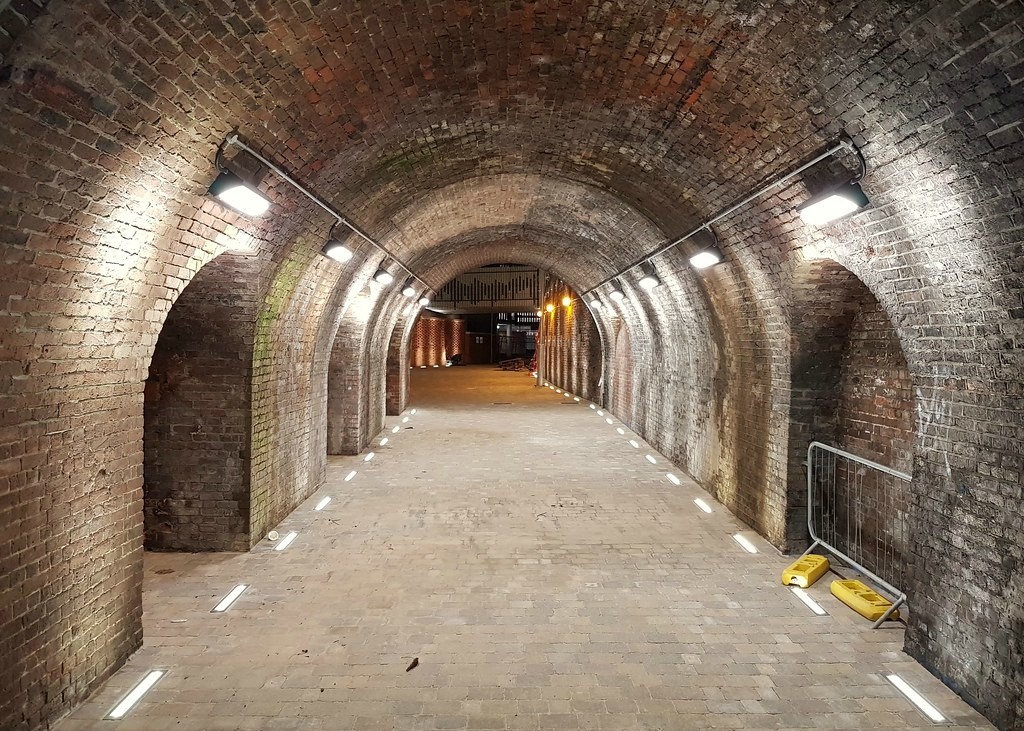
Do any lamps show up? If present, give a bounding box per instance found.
[207,169,274,220]
[794,180,870,226]
[373,268,394,285]
[417,294,429,305]
[609,290,626,301]
[637,274,661,290]
[321,239,354,263]
[689,244,724,268]
[400,285,416,297]
